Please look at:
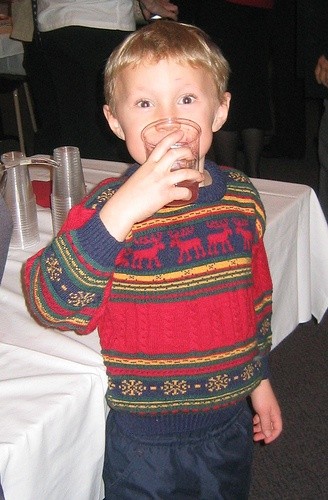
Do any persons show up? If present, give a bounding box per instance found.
[20,22,284,500]
[0,0,328,198]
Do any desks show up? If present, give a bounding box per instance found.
[0,12,39,158]
[0,154,328,500]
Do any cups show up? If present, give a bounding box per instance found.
[1,152,40,248]
[51,146,87,237]
[140,118,202,207]
